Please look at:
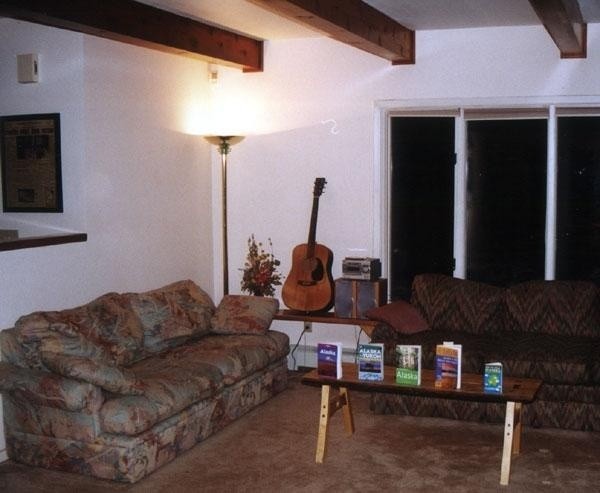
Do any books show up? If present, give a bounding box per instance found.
[484,361,503,393]
[317,342,343,379]
[435,343,462,389]
[396,345,422,387]
[358,343,384,381]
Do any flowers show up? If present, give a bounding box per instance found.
[240,232,285,297]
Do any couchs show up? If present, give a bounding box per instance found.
[0,278,291,486]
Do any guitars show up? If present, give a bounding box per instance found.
[281,177,335,313]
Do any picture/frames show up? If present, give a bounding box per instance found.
[1,112,64,213]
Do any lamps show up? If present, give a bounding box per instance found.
[205,135,246,297]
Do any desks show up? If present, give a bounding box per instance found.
[271,311,384,328]
[300,361,540,488]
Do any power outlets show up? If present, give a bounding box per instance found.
[303,321,312,333]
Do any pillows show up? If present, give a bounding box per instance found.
[209,294,278,335]
[364,299,431,338]
[370,270,600,432]
[38,349,144,396]
